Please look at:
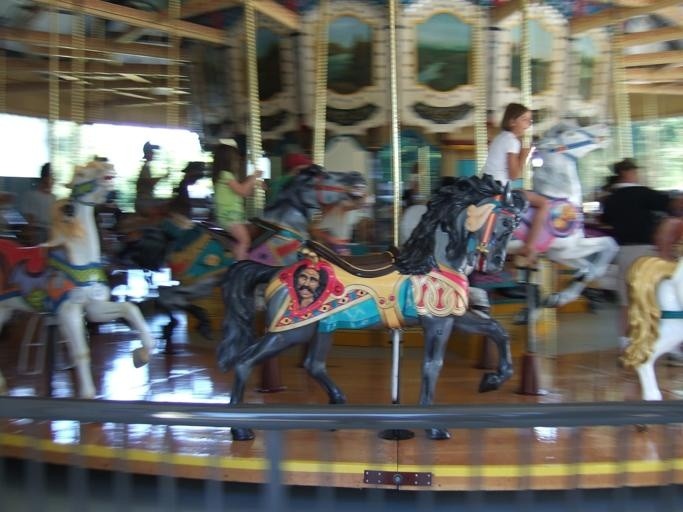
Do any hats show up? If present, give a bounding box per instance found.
[144,142,159,151]
[284,155,309,168]
[615,159,637,171]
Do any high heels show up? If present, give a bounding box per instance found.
[514,254,539,271]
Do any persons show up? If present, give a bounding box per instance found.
[134,142,169,216]
[215,145,265,262]
[477,102,550,266]
